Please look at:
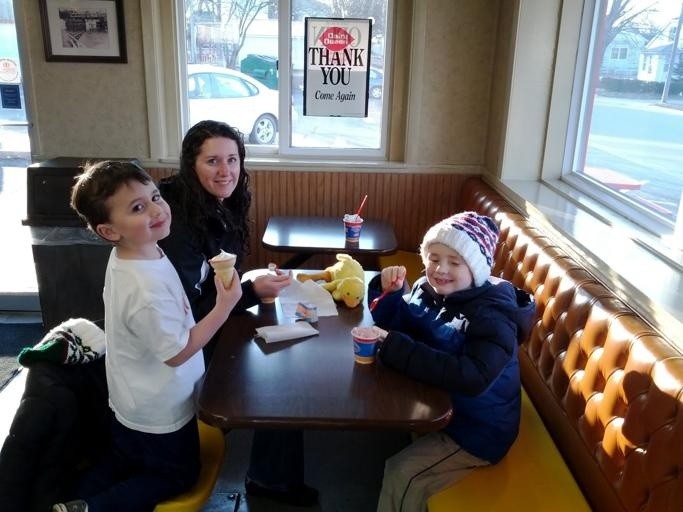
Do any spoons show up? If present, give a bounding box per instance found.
[370,277,399,313]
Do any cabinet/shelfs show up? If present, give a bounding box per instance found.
[28,156,140,332]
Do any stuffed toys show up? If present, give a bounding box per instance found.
[297,253,365,308]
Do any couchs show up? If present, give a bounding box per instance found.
[380,176,683,512]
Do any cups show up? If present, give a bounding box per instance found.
[351,326,381,365]
[343,215,364,243]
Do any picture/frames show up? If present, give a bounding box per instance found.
[41,0,128,63]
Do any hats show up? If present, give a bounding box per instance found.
[420,211,499,287]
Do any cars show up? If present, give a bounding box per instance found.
[188,64,294,145]
[369,68,383,98]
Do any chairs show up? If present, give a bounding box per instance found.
[33,319,226,511]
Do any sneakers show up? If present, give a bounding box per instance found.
[52,499,89,512]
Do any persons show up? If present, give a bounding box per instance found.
[53,160,241,512]
[368,212,535,512]
[159,121,322,508]
